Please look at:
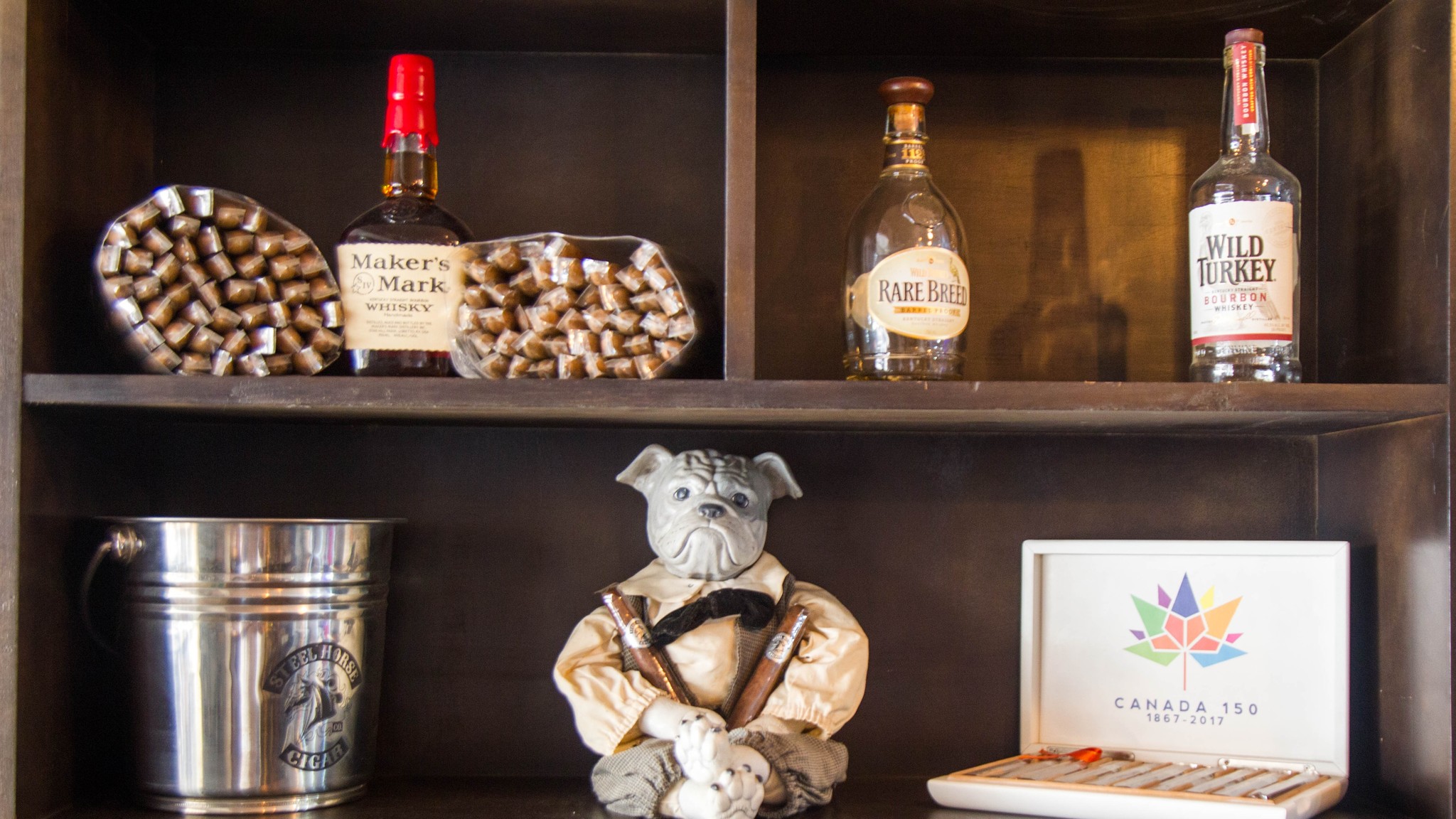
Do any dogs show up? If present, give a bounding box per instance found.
[552,444,869,819]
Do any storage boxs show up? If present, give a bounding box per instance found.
[928,538,1354,818]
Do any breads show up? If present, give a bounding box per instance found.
[601,588,690,705]
[96,186,344,382]
[457,237,695,382]
[726,604,809,731]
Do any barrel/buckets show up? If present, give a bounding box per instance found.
[75,517,409,814]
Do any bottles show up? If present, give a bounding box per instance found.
[335,55,475,377]
[843,78,971,378]
[1189,29,1304,384]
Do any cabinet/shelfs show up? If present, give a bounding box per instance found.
[0,1,1456,819]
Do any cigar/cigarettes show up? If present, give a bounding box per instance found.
[603,586,691,706]
[725,604,811,733]
